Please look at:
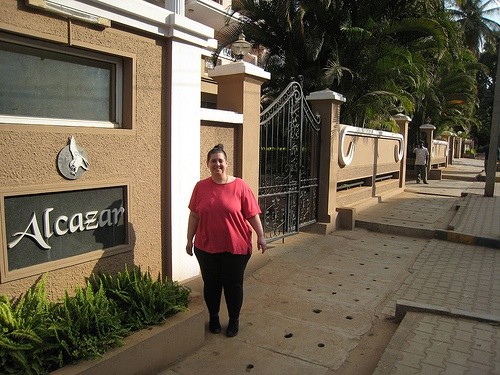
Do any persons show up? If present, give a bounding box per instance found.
[185,143,268,338]
[410,141,430,184]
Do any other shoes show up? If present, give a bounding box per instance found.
[424,183,428,184]
[226,319,239,337]
[417,182,421,184]
[209,316,220,333]
